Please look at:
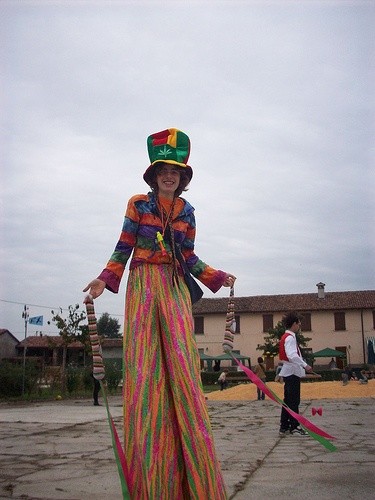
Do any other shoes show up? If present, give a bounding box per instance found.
[93,403,99,406]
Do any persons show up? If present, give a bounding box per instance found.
[330,359,369,385]
[214,357,284,400]
[92,372,103,406]
[83,128,237,500]
[279,310,314,437]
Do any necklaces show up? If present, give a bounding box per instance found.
[155,195,174,255]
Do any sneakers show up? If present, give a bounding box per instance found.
[289,426,310,436]
[279,427,290,436]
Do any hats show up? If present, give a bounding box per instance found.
[143,128,193,189]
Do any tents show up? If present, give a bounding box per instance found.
[314,348,346,370]
[199,351,252,371]
